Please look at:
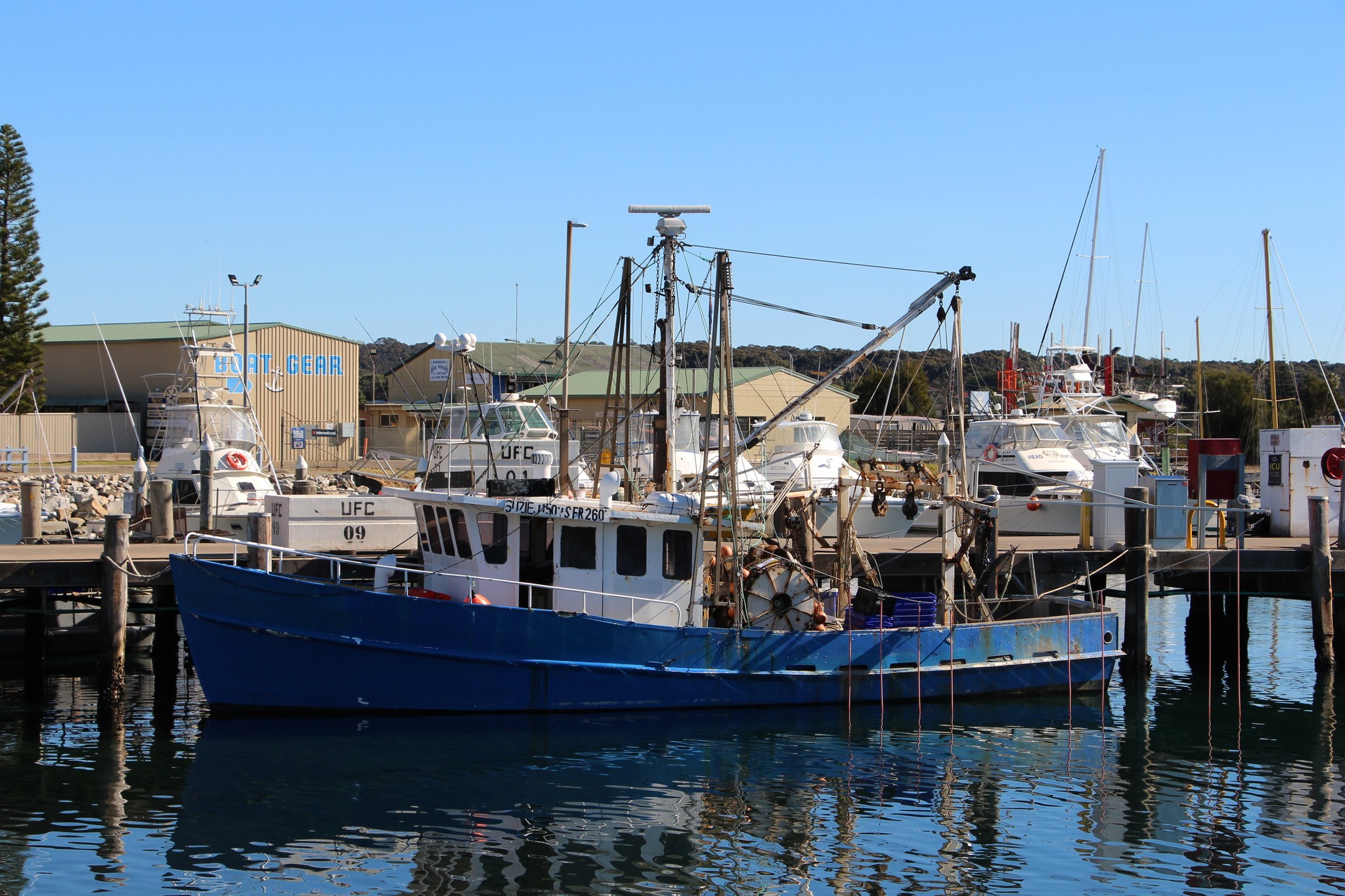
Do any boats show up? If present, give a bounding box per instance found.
[0,368,59,544]
[94,296,287,538]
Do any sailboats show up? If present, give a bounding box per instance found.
[169,144,1191,724]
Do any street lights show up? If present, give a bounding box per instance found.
[563,219,589,410]
[227,274,264,405]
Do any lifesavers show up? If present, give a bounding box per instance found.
[226,450,248,470]
[984,443,998,463]
